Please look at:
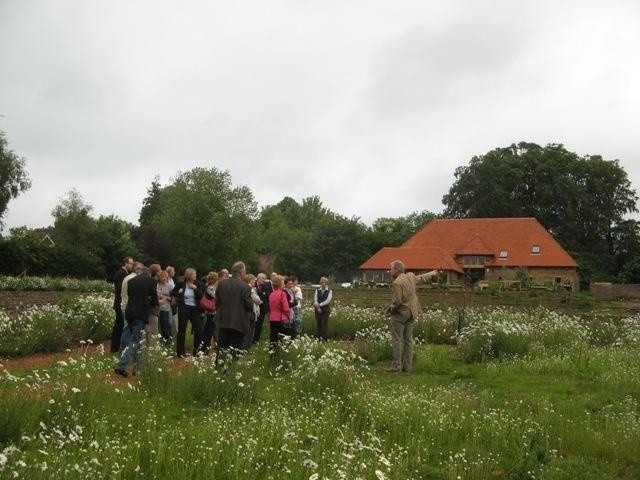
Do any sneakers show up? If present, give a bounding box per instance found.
[114,366,129,378]
[384,367,411,373]
[253,339,258,344]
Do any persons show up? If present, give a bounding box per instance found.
[384,260,440,373]
[108,256,335,378]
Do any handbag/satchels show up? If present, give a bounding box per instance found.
[199,284,217,313]
[280,318,294,330]
